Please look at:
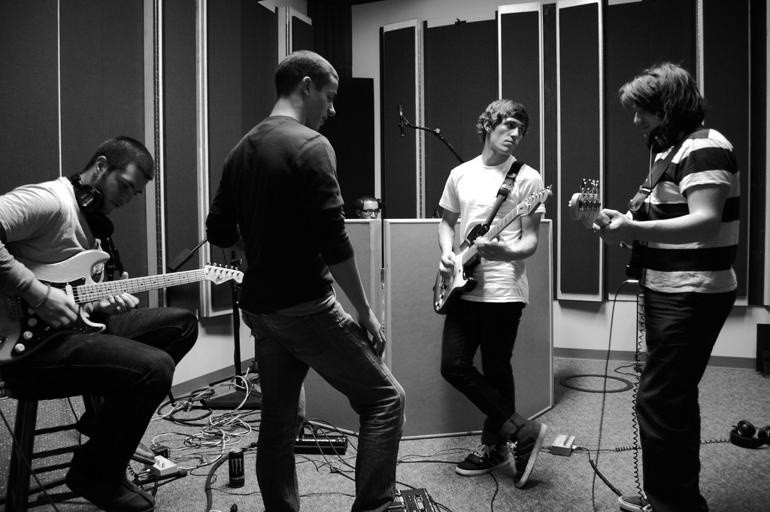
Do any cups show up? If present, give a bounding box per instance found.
[229,448,245,488]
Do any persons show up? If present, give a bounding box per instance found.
[588,60,742,510]
[353,194,381,219]
[0,136,200,510]
[206,51,409,511]
[435,100,548,487]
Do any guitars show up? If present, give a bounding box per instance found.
[568,178,649,281]
[433,184,554,314]
[0,248,244,362]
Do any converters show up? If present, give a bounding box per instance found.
[552,435,576,455]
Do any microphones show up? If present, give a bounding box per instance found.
[398,103,406,136]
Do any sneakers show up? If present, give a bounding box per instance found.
[617,495,653,512]
[73,411,155,466]
[65,472,155,512]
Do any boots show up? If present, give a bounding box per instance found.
[455,444,511,476]
[501,412,548,488]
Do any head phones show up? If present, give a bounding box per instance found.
[355,197,383,210]
[730,420,770,449]
[648,123,678,153]
[69,173,116,238]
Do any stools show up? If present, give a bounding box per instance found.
[1,378,104,511]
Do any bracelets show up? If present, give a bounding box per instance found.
[32,283,50,309]
